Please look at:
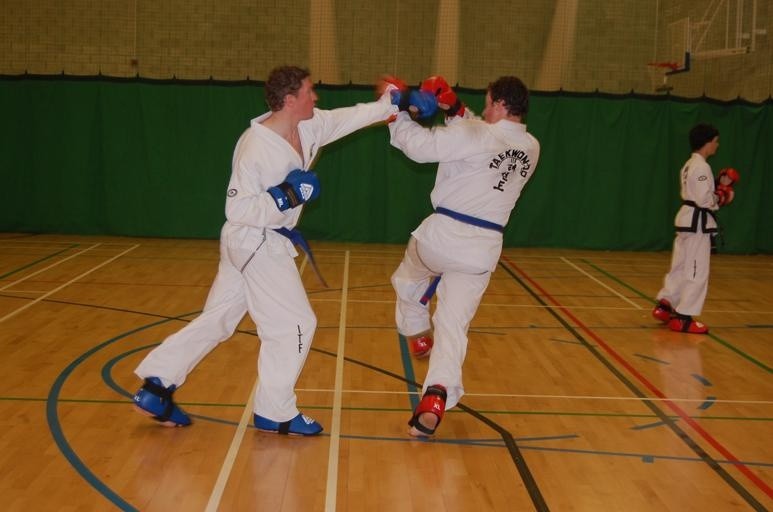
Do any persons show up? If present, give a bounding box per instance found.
[651,124,739,334]
[376,74,542,438]
[131,66,437,436]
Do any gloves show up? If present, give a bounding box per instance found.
[420,75,465,119]
[389,88,437,118]
[716,169,739,188]
[377,78,406,123]
[266,168,320,212]
[714,184,735,206]
